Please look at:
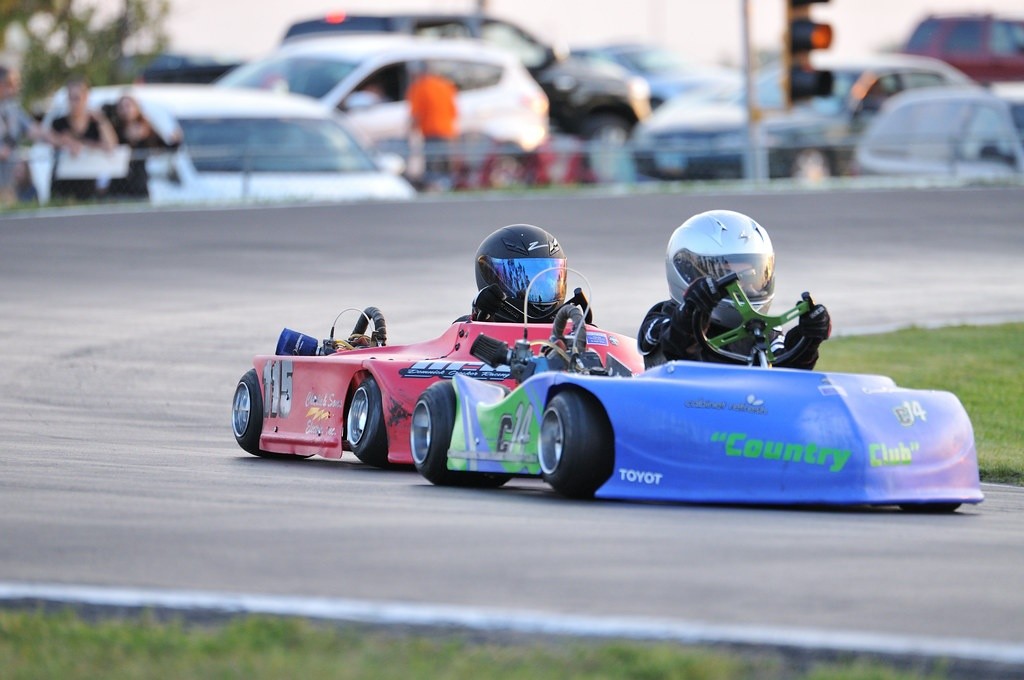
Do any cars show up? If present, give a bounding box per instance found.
[206,29,551,158]
[903,15,1023,86]
[25,77,418,208]
[620,45,1021,180]
[281,9,652,143]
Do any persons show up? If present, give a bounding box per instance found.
[407,59,468,180]
[451,222,592,324]
[0,63,184,205]
[636,210,835,372]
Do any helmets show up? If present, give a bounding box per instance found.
[475,224,567,324]
[665,210,775,327]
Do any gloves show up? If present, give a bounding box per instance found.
[796,301,832,340]
[682,275,729,314]
[474,283,508,317]
